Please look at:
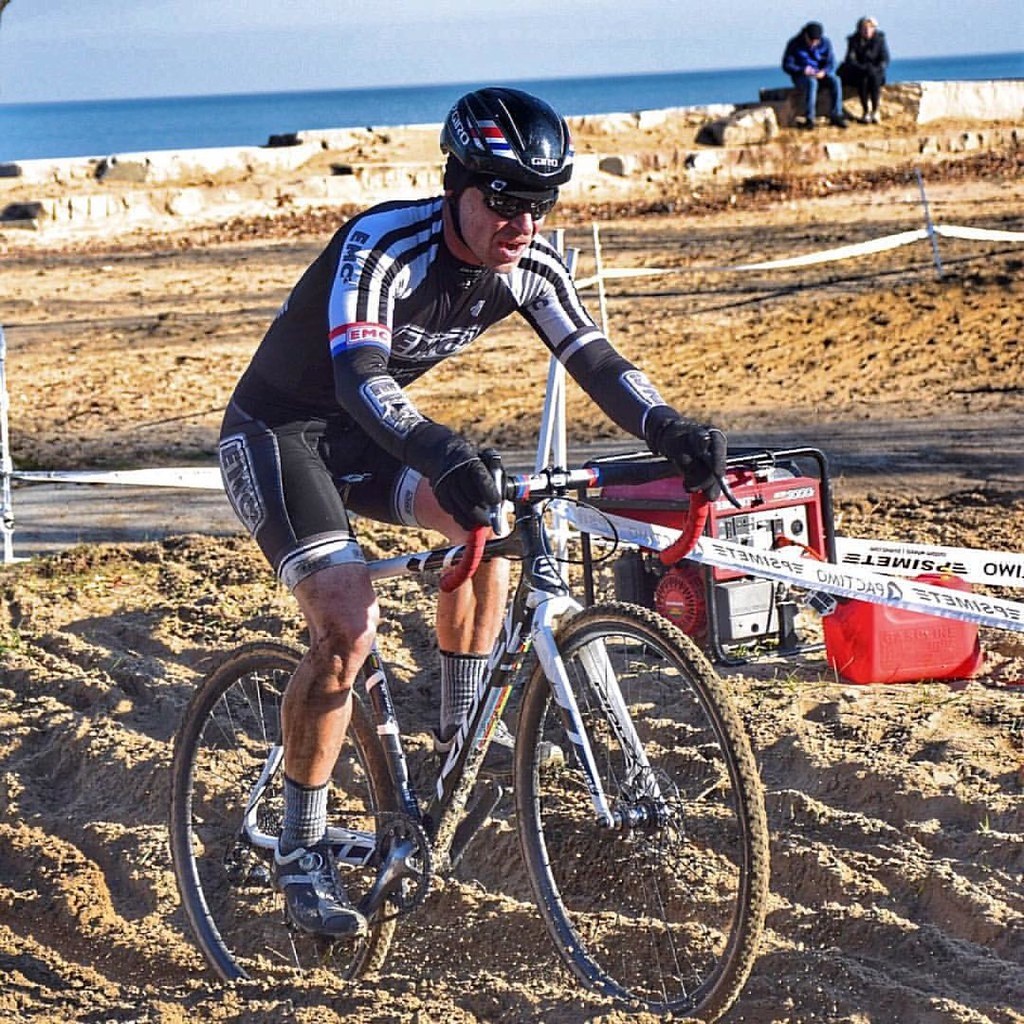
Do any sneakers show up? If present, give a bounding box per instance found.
[427,716,564,779]
[272,836,369,940]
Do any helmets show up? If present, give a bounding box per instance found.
[439,86,575,193]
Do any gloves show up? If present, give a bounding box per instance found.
[643,406,729,501]
[403,423,501,531]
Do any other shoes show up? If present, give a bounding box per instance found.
[862,113,873,123]
[871,110,881,124]
[805,118,819,133]
[830,116,847,128]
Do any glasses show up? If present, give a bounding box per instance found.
[475,185,558,220]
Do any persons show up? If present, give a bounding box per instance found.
[781,22,850,129]
[846,15,892,125]
[217,87,725,936]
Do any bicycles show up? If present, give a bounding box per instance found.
[165,457,774,1024]
